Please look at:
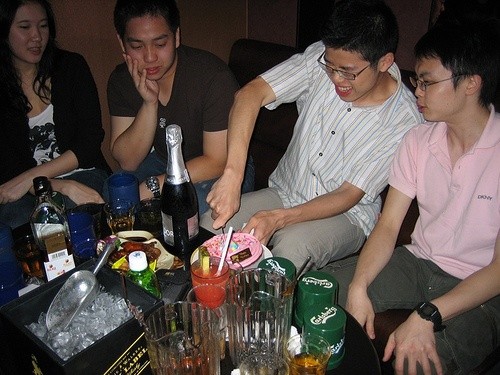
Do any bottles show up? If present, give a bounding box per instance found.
[125,251,162,300]
[29,176,80,284]
[159,124,201,260]
[11,222,47,281]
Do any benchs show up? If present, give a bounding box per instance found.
[229,38,420,352]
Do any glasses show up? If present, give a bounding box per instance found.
[409,73,474,92]
[317,49,386,81]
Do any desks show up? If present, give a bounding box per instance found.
[134,224,380,375]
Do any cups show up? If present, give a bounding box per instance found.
[182,283,228,358]
[107,173,140,210]
[104,199,135,234]
[191,256,230,302]
[0,261,26,305]
[144,301,221,375]
[240,352,289,375]
[67,213,97,258]
[72,204,103,239]
[136,197,161,236]
[226,268,294,369]
[284,333,331,375]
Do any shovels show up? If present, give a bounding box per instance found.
[46,244,113,334]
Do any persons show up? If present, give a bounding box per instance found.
[0,0,117,240]
[198,0,427,280]
[316,18,500,375]
[102,0,255,218]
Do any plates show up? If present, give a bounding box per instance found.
[116,231,154,243]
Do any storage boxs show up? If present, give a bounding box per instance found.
[0,259,165,375]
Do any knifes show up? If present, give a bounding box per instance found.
[221,227,227,235]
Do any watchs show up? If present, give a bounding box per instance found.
[415,301,444,332]
[145,176,160,197]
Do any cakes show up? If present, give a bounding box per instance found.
[199,233,265,281]
